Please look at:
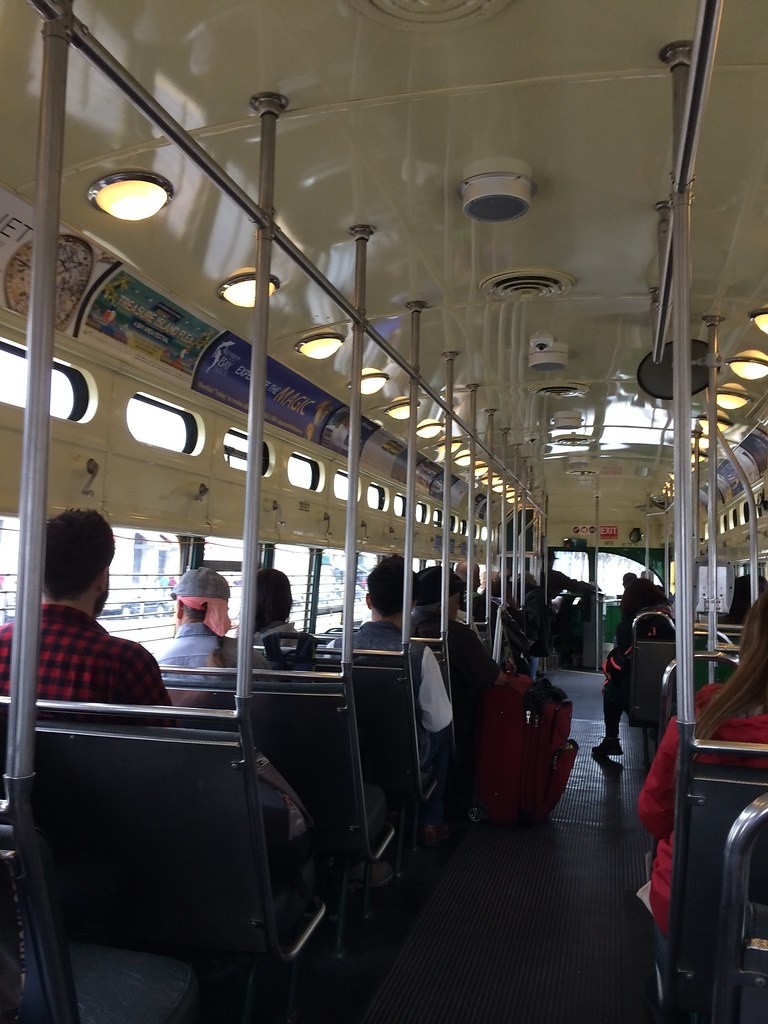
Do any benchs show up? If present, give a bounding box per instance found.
[0,583,543,1024]
[627,608,768,1024]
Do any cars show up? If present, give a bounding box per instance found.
[5,601,175,623]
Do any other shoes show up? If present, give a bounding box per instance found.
[561,663,584,671]
[643,970,678,1024]
[592,737,623,756]
[348,861,393,891]
[420,821,443,845]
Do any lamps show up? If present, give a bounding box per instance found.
[434,439,522,504]
[697,410,732,435]
[216,268,281,309]
[293,333,344,360]
[87,171,174,221]
[726,350,768,380]
[717,382,751,410]
[348,373,389,396]
[749,308,768,334]
[691,435,709,472]
[417,419,444,438]
[385,395,422,421]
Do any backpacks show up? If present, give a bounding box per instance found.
[255,748,316,929]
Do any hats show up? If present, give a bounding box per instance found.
[170,567,230,601]
[413,566,465,606]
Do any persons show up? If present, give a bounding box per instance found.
[169,577,176,591]
[0,508,182,729]
[636,587,768,1024]
[717,574,768,639]
[540,550,602,615]
[455,560,552,681]
[160,576,169,592]
[323,555,452,848]
[592,573,675,756]
[153,567,396,895]
[410,567,505,811]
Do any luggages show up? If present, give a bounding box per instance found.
[468,674,579,831]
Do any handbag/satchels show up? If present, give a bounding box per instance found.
[602,650,628,682]
